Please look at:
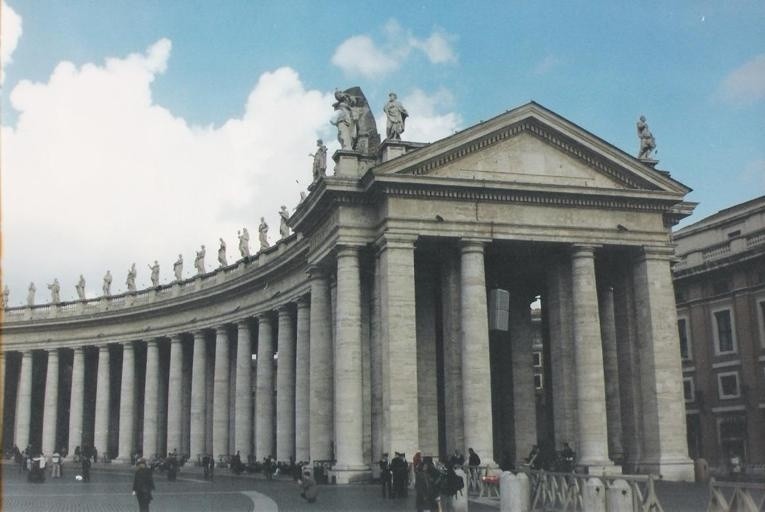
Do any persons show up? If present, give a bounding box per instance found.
[257,217,271,249]
[0,436,99,483]
[238,227,250,256]
[257,452,318,502]
[526,443,573,488]
[379,446,481,512]
[331,91,364,150]
[278,205,291,238]
[311,138,329,181]
[637,116,656,160]
[730,449,741,472]
[102,270,112,295]
[126,263,136,291]
[26,281,37,307]
[2,284,9,310]
[75,275,86,298]
[148,260,160,286]
[195,245,206,273]
[217,238,228,267]
[130,448,245,512]
[383,93,410,140]
[173,254,183,280]
[47,277,62,302]
